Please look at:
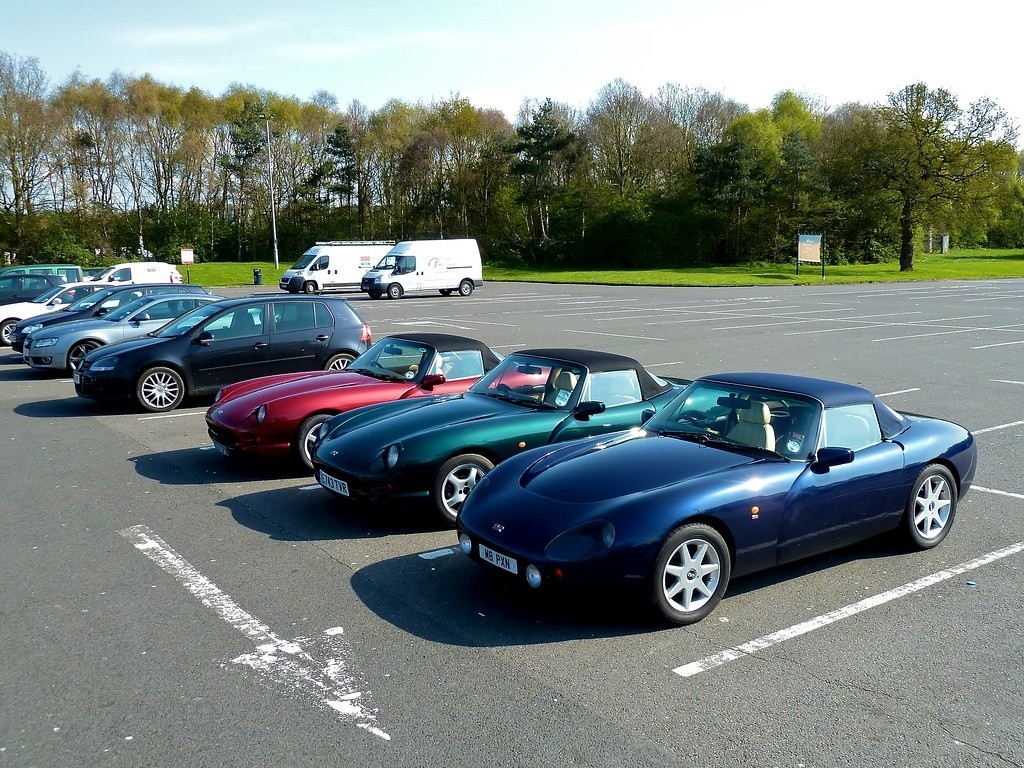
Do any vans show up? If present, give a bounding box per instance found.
[361,239,483,299]
[0,264,84,283]
[279,240,397,295]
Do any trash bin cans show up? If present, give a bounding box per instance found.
[253,268,262,285]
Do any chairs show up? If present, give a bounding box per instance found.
[544,371,578,408]
[228,310,254,336]
[725,401,776,453]
[602,375,638,408]
[827,415,872,450]
[1,280,191,317]
[430,354,444,376]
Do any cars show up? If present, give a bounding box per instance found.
[0,275,68,304]
[22,294,282,377]
[0,281,143,345]
[88,262,183,283]
[72,294,373,411]
[9,283,210,354]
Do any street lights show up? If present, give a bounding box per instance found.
[258,113,279,269]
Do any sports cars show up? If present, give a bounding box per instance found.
[310,348,739,526]
[205,334,553,474]
[455,373,977,628]
